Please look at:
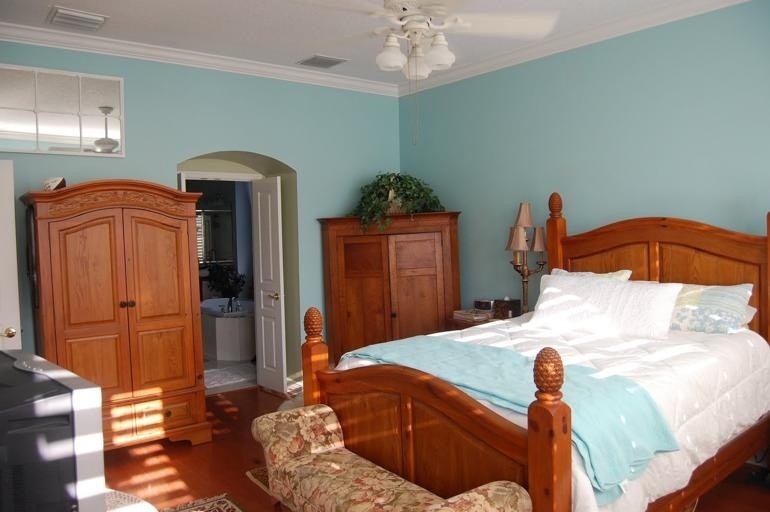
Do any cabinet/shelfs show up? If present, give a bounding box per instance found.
[315,209,466,369]
[18,178,216,450]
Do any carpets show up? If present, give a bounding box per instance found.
[158,489,256,511]
[201,361,258,390]
[246,465,272,498]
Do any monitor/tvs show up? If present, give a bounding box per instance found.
[0,349,107,512]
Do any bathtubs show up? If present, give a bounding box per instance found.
[201,298,256,361]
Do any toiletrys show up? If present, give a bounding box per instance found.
[236,300,240,312]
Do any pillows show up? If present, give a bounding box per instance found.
[664,282,755,336]
[548,266,635,282]
[520,272,686,340]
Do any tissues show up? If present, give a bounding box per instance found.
[493,296,521,319]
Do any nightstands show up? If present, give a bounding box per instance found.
[445,316,493,331]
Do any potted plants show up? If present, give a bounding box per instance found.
[343,170,448,235]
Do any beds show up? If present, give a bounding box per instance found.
[300,190,770,512]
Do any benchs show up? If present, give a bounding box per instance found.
[250,401,536,512]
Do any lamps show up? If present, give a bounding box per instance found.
[94,103,120,158]
[370,1,460,83]
[502,195,548,317]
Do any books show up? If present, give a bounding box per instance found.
[454,309,494,323]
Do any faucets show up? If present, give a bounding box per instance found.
[228,296,236,312]
